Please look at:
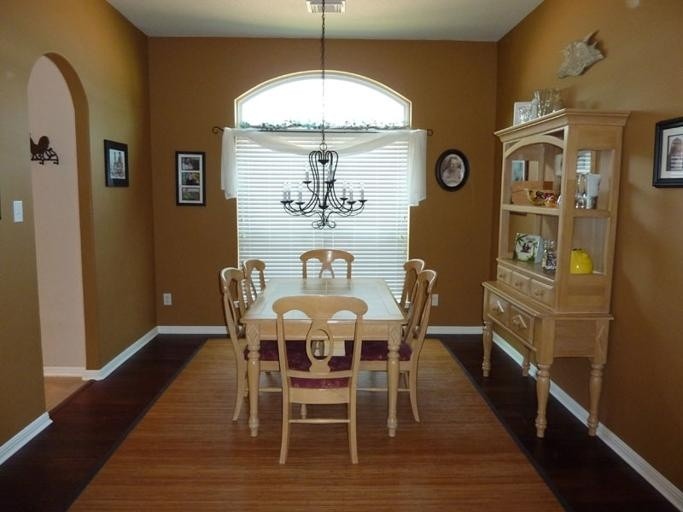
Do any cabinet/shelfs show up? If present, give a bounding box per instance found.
[481,279,615,439]
[481,107,631,312]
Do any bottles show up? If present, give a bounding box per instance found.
[542,241,557,274]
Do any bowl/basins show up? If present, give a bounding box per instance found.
[526,188,553,206]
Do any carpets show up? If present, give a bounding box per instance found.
[66,338,572,511]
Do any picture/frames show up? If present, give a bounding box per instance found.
[512,160,526,216]
[652,117,682,188]
[513,101,531,125]
[175,151,206,206]
[104,139,129,187]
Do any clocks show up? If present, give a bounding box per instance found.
[436,149,470,192]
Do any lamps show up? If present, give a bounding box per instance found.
[280,2,367,230]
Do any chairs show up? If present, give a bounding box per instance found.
[219,267,308,423]
[300,249,354,279]
[273,296,367,464]
[399,258,425,320]
[357,270,438,423]
[242,260,266,309]
